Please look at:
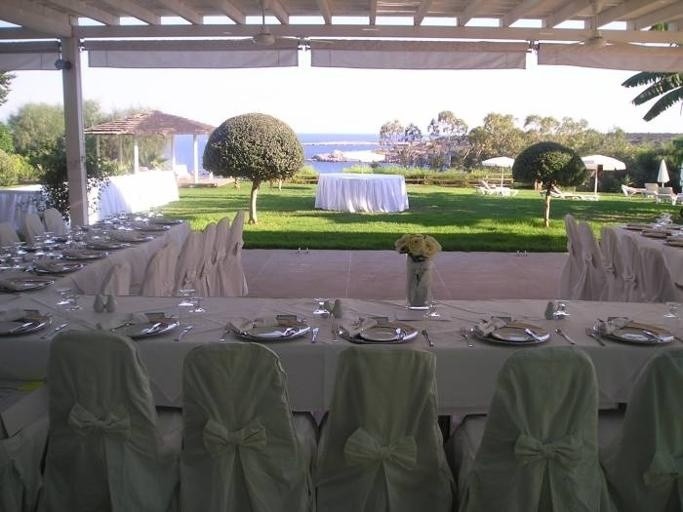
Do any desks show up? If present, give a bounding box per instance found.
[2,169,178,223]
[2,210,183,291]
[315,174,410,215]
[3,298,683,440]
[608,218,682,293]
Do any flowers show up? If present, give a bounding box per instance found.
[393,232,443,287]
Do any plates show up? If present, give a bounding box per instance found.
[235,314,312,343]
[594,316,676,349]
[614,209,683,254]
[348,317,420,346]
[116,315,180,341]
[1,207,196,294]
[471,318,551,345]
[3,311,51,338]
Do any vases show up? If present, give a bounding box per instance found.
[405,255,434,311]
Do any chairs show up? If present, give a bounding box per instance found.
[178,228,204,295]
[562,214,578,298]
[454,347,605,512]
[42,206,70,238]
[21,213,45,241]
[656,186,676,206]
[636,246,672,305]
[603,347,683,512]
[177,343,311,511]
[0,399,48,512]
[676,192,683,202]
[44,330,177,512]
[644,183,658,196]
[599,226,619,298]
[164,242,181,294]
[141,254,170,299]
[198,221,218,295]
[100,261,135,298]
[1,222,22,244]
[620,184,648,200]
[620,233,636,300]
[233,209,248,297]
[310,345,452,511]
[218,216,231,297]
[576,220,599,298]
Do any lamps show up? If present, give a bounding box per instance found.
[78,46,90,71]
[295,34,311,72]
[525,37,542,75]
